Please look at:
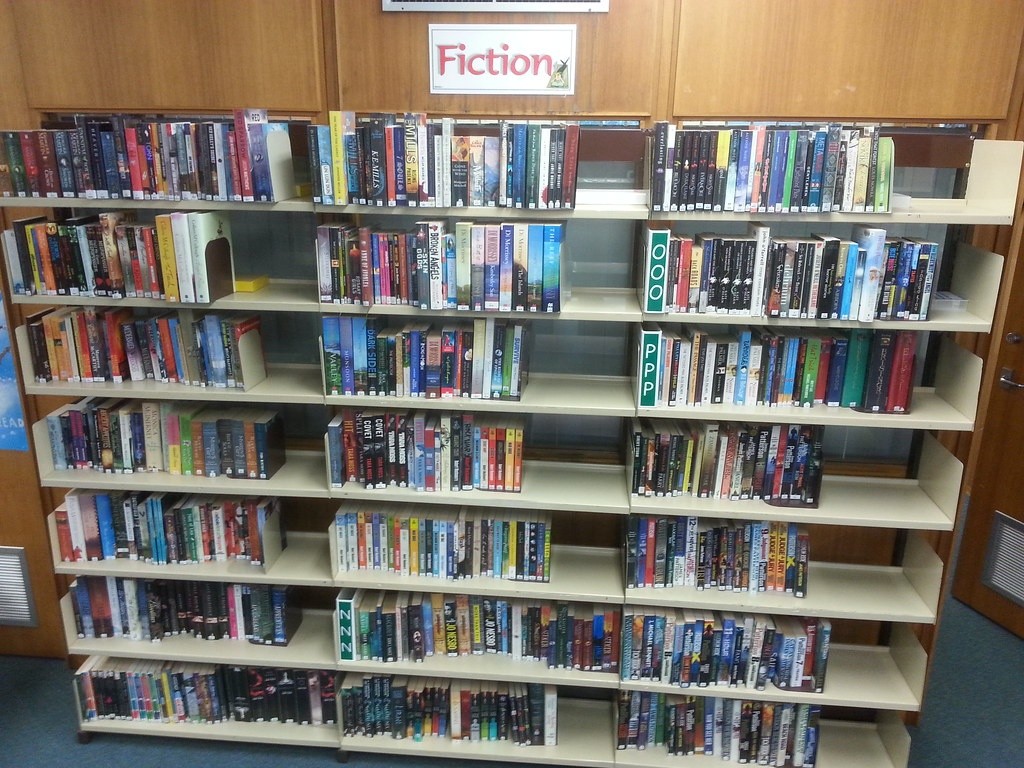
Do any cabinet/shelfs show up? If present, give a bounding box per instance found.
[0,137,1024,768]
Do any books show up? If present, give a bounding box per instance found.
[0,106,937,768]
